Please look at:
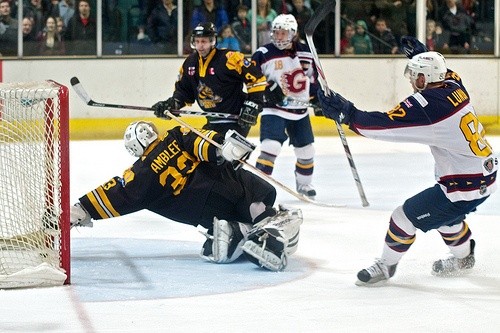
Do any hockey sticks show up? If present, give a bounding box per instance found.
[72,76,239,120]
[303,0,369,207]
[288,98,319,108]
[164,111,349,208]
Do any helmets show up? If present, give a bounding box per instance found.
[124,121,160,157]
[192,22,217,38]
[269,13,297,49]
[405,51,446,89]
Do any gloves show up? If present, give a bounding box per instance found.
[239,99,263,127]
[264,79,287,106]
[316,88,353,124]
[400,35,428,58]
[151,97,185,118]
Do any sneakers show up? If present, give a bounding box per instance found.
[432,240,475,276]
[296,183,316,202]
[211,217,234,261]
[355,261,397,285]
[240,238,283,271]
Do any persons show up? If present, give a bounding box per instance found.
[341,0,416,54]
[250,14,322,198]
[46,121,303,272]
[192,0,313,52]
[147,0,187,41]
[427,0,494,54]
[315,35,498,287]
[0,0,97,57]
[152,21,265,140]
